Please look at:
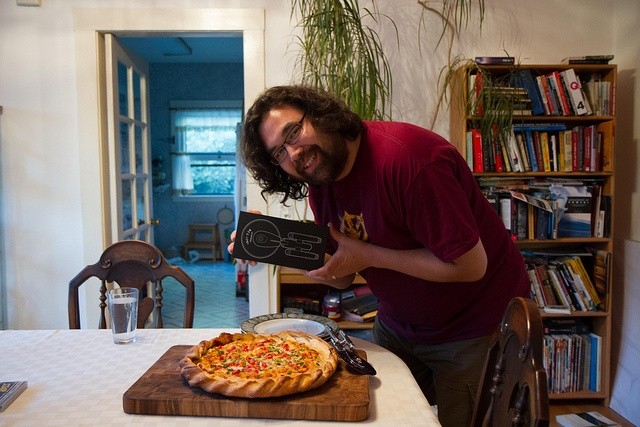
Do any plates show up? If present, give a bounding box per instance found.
[240,311,340,341]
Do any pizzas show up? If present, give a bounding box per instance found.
[179,330,338,398]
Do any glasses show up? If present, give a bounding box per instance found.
[268,112,307,166]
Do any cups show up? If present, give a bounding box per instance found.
[107,286,139,346]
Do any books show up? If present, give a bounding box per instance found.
[479,179,612,239]
[590,338,597,392]
[466,132,473,174]
[475,56,515,65]
[472,129,483,174]
[469,67,614,120]
[570,60,609,64]
[485,122,605,173]
[542,316,591,394]
[567,54,615,60]
[521,248,605,312]
[596,338,602,392]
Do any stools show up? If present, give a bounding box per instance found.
[186,223,220,261]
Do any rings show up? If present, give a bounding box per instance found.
[331,274,337,280]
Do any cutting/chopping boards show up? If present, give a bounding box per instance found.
[123,345,370,422]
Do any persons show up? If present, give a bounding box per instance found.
[226,85,532,426]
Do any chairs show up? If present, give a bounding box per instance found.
[68,240,194,329]
[469,297,550,426]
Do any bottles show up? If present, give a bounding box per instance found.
[323,295,340,321]
[292,295,320,315]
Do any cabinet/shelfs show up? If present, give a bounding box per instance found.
[449,65,618,408]
[277,264,378,329]
